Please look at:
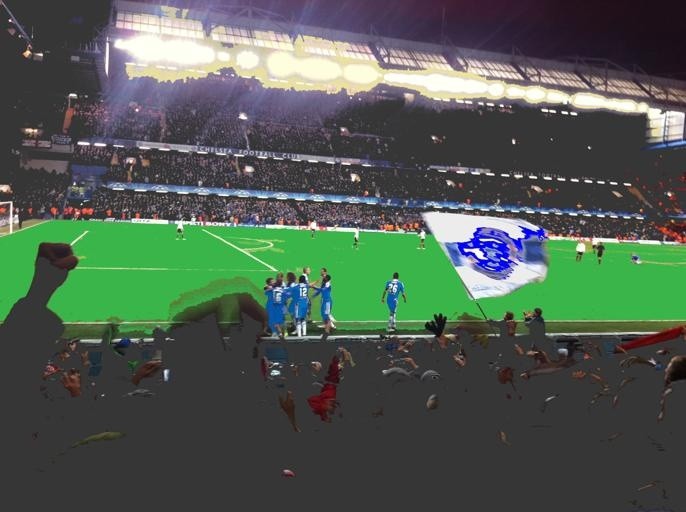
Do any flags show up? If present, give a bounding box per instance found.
[418,204,552,300]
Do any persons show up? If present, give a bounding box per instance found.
[629,251,644,265]
[265,272,288,338]
[520,307,547,337]
[281,272,298,338]
[295,275,320,338]
[1,313,686,512]
[574,240,587,260]
[2,50,686,244]
[415,227,425,249]
[0,241,79,399]
[381,271,409,335]
[351,224,360,251]
[484,310,518,339]
[311,275,334,338]
[264,277,279,339]
[595,242,605,264]
[302,266,313,325]
[590,233,598,255]
[317,268,337,330]
